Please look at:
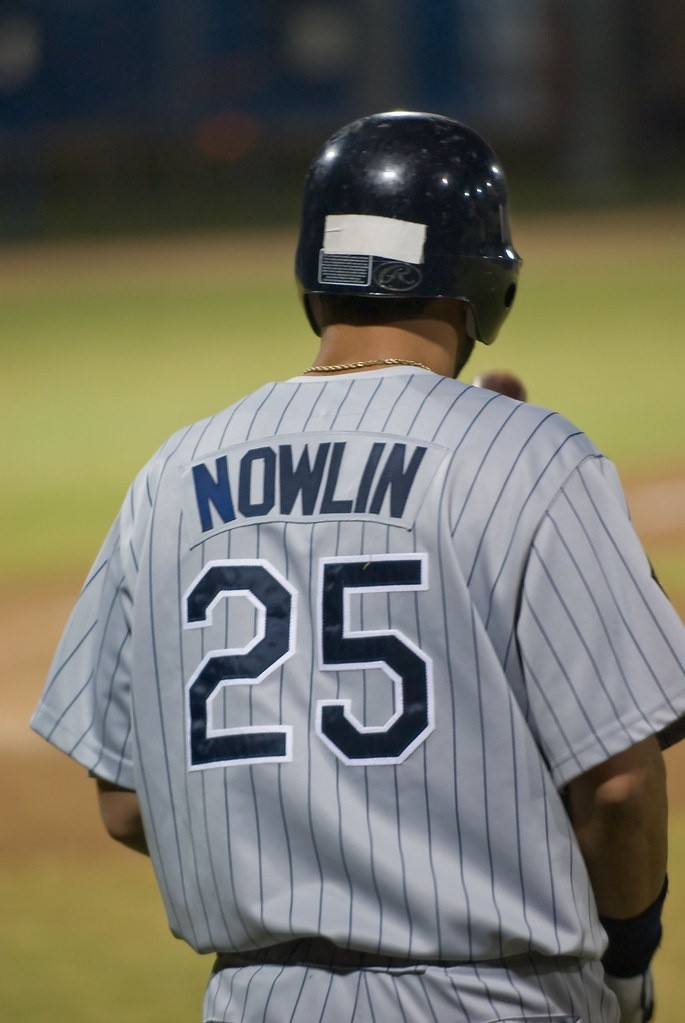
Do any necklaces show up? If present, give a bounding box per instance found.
[304,359,432,374]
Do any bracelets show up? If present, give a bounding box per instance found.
[599,873,667,979]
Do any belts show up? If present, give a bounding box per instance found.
[217,937,414,970]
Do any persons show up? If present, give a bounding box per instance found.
[28,110,684,1023]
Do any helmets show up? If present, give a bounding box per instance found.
[292,110,523,345]
